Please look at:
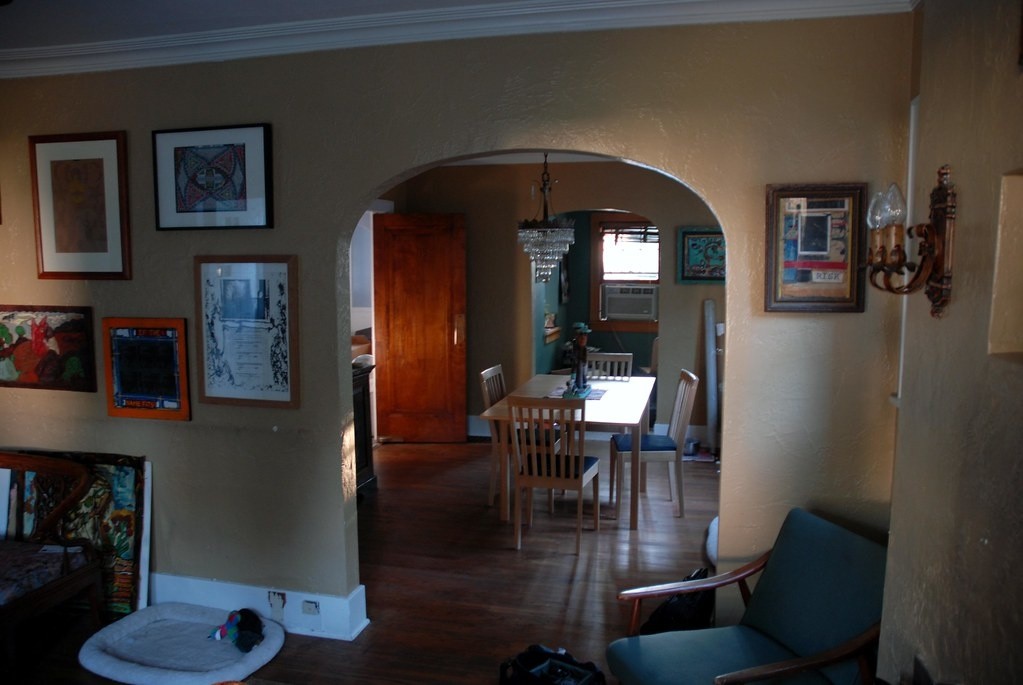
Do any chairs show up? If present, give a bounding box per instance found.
[0,453,103,670]
[480,364,566,513]
[610,369,699,520]
[607,510,888,685]
[561,353,633,502]
[507,396,600,556]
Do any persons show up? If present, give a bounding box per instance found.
[558,322,597,370]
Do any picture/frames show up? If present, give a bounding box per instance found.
[101,317,191,421]
[559,254,571,304]
[28,130,133,280]
[0,448,146,618]
[0,305,97,393]
[677,226,726,284]
[151,123,273,231]
[764,182,868,313]
[194,254,299,409]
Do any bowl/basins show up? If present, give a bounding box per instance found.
[684,439,700,455]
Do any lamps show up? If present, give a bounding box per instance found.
[518,152,575,282]
[867,163,957,318]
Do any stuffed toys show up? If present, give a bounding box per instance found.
[207,608,263,653]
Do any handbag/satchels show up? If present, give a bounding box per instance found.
[499,641,606,685]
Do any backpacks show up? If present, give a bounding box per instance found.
[640,567,715,635]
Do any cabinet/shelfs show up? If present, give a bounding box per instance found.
[352,364,378,504]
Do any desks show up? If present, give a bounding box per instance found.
[479,374,657,528]
